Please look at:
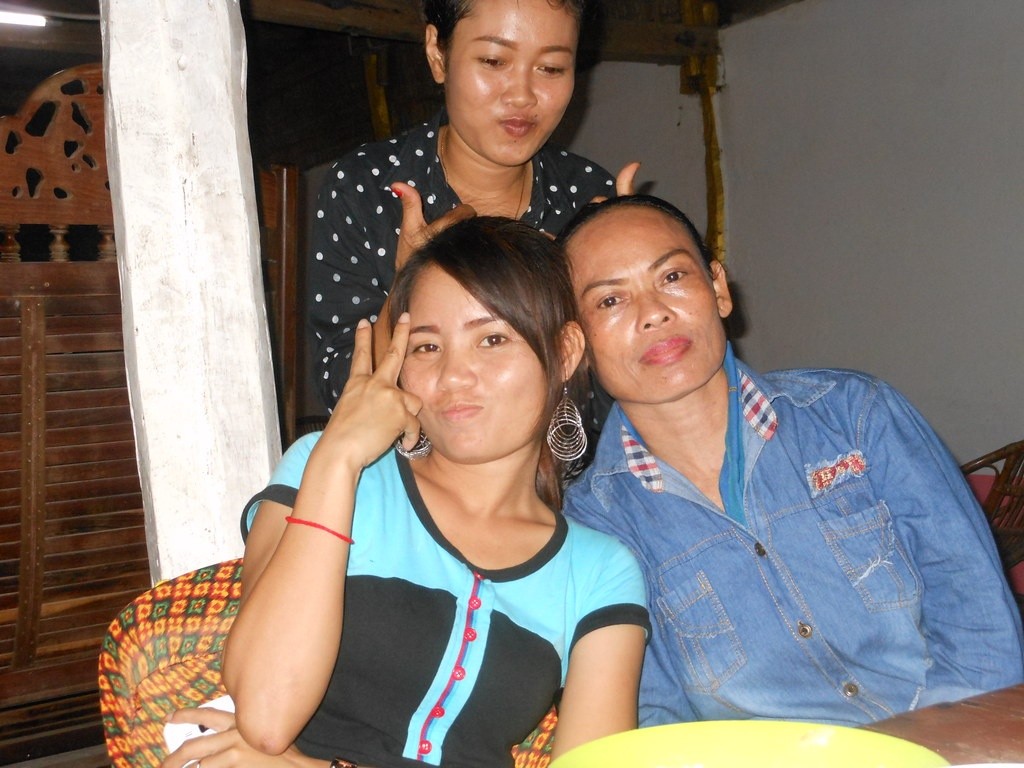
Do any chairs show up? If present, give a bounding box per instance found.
[98,555,559,768]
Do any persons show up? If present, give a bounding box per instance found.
[559,196,1023,729]
[162,216,652,768]
[312,0,640,502]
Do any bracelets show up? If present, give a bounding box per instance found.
[330,758,358,768]
[285,515,354,544]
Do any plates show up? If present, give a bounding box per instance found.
[546,719,950,768]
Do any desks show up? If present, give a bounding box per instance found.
[852,682,1024,768]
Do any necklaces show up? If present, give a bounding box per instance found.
[441,126,527,218]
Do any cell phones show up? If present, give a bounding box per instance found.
[164,695,236,768]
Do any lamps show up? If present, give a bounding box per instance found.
[0,11,64,27]
